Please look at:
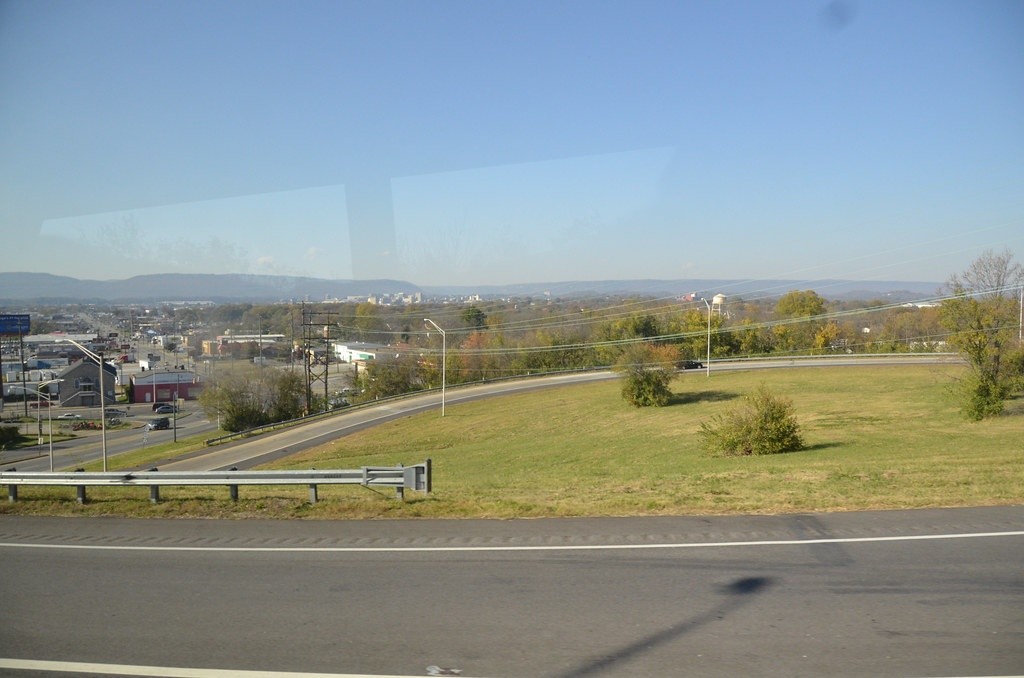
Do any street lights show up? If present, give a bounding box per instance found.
[423,318,445,417]
[38,378,65,441]
[700,297,710,377]
[7,385,54,472]
[54,338,107,474]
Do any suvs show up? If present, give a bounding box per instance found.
[147,417,168,431]
[152,403,177,411]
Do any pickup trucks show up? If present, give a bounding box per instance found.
[30,401,53,409]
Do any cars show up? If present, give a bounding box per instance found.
[57,412,82,420]
[105,408,127,418]
[678,360,702,370]
[155,405,178,414]
